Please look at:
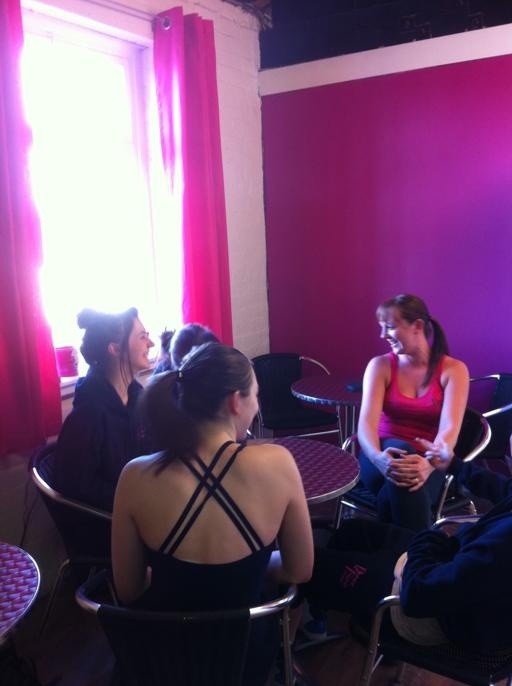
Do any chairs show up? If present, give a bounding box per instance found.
[468,372,512,459]
[362,515,512,684]
[334,407,493,531]
[78,566,298,683]
[27,438,113,628]
[249,351,335,439]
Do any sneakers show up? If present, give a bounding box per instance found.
[300,610,327,642]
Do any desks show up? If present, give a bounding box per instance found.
[269,437,363,505]
[291,375,362,456]
[0,541,40,637]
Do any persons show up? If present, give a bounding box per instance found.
[53,308,154,569]
[154,331,175,374]
[153,324,219,375]
[355,294,470,666]
[279,437,512,641]
[110,341,315,686]
